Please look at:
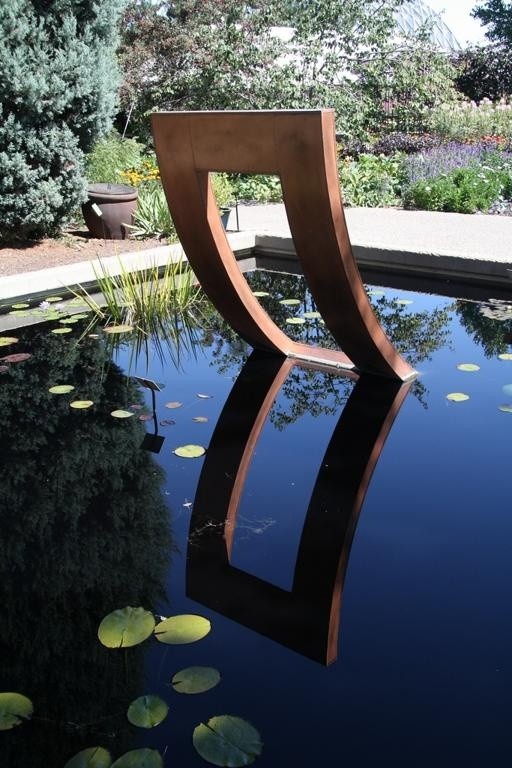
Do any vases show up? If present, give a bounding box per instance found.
[219,209,231,231]
